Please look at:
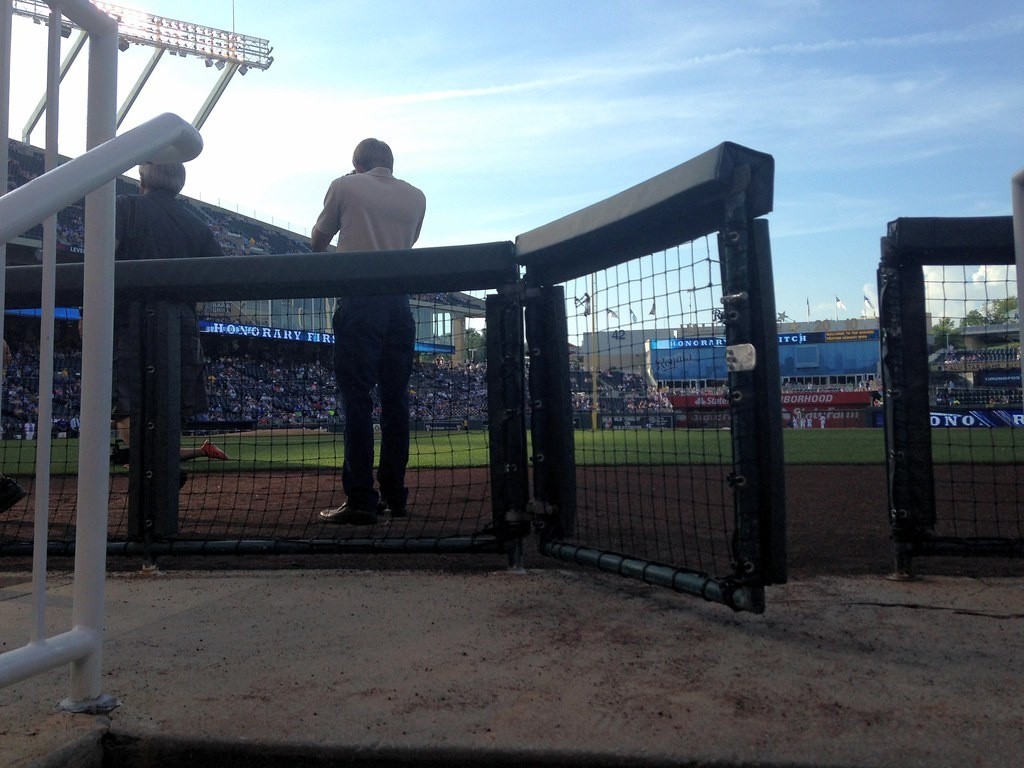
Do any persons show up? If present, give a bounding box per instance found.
[103,157,230,463]
[310,138,426,525]
[0,141,1024,512]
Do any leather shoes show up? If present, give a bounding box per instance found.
[374,488,407,517]
[318,502,378,525]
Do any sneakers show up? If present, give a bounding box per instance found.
[201,439,230,461]
